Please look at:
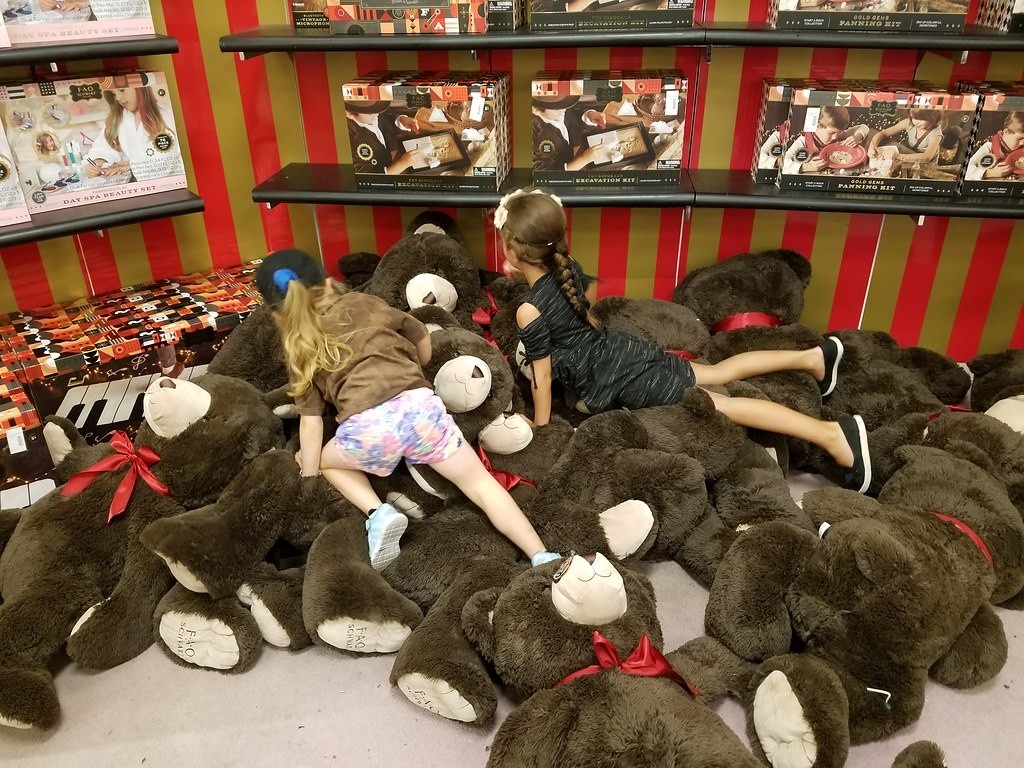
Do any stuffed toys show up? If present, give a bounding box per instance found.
[1,210,1024,768]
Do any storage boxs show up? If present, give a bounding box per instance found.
[531,70,690,186]
[0,1,155,47]
[341,69,512,192]
[765,0,1024,34]
[286,0,695,36]
[751,77,1024,200]
[0,67,189,228]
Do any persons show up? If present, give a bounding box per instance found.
[493,189,872,494]
[8,84,186,182]
[39,0,90,14]
[964,111,1024,182]
[254,247,564,574]
[345,101,422,174]
[757,104,945,175]
[531,95,607,171]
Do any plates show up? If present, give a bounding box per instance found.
[1005,148,1024,174]
[820,141,868,168]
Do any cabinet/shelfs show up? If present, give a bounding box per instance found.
[219,21,1024,229]
[0,34,205,247]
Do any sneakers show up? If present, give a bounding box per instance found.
[531,551,562,567]
[838,414,872,493]
[364,503,408,572]
[819,336,844,396]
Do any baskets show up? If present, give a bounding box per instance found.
[633,94,680,123]
[442,101,487,129]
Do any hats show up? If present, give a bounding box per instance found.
[533,95,581,110]
[256,249,324,306]
[346,100,390,114]
